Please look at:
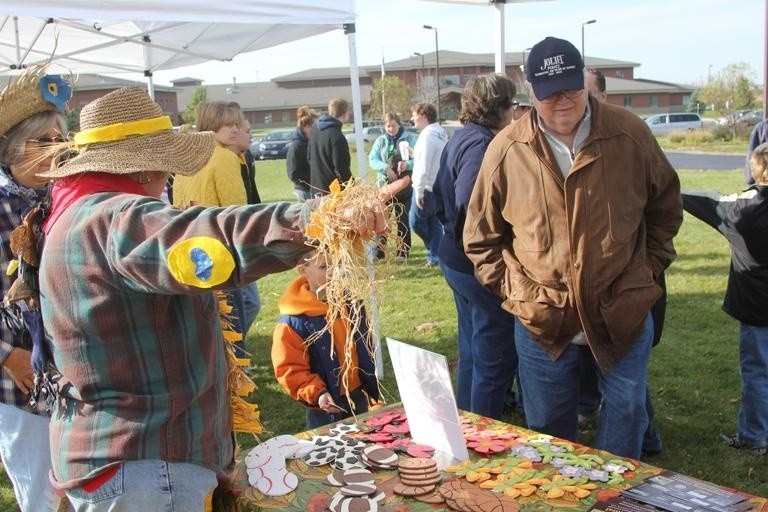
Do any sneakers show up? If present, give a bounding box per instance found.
[730,434,766,454]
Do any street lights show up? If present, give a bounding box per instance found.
[521,48,533,93]
[424,24,441,126]
[414,52,426,104]
[581,20,597,66]
[706,62,713,82]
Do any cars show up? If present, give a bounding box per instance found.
[257,128,296,159]
[249,137,259,159]
[716,108,763,129]
[172,125,197,135]
[702,118,719,133]
[353,121,420,135]
[344,125,416,144]
[369,117,415,128]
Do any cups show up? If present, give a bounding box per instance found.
[398,140,411,161]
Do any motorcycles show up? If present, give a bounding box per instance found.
[712,122,734,142]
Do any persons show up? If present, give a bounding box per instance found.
[464,37,683,462]
[745,119,768,186]
[0,63,79,512]
[287,106,321,202]
[369,111,417,262]
[578,269,667,453]
[167,100,263,380]
[271,249,379,431]
[581,68,606,103]
[433,72,520,417]
[680,142,768,458]
[412,102,449,267]
[307,97,356,199]
[32,84,411,512]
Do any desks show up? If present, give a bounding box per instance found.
[220,399,767,511]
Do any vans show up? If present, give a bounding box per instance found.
[644,112,703,138]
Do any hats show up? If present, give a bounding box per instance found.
[526,38,585,101]
[0,61,73,133]
[35,86,216,178]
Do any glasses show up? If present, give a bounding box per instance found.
[28,134,69,151]
